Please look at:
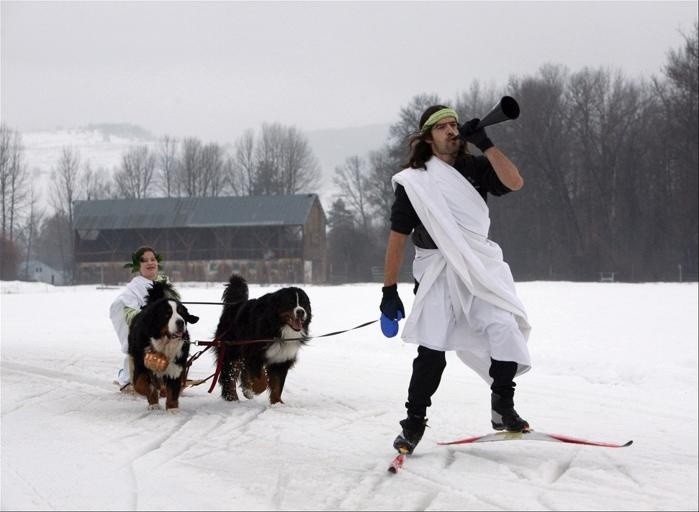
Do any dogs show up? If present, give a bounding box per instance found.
[127,279,200,415]
[210,273,312,406]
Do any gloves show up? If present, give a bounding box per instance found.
[457,117,494,153]
[380,282,405,321]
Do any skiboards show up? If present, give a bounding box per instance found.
[388,428,633,474]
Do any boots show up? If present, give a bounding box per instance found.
[393,410,428,455]
[490,392,529,432]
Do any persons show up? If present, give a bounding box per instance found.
[379,103,531,454]
[109,245,179,393]
